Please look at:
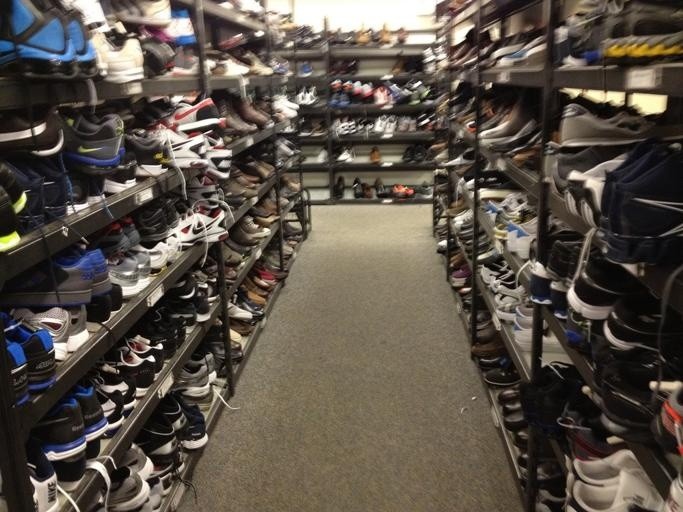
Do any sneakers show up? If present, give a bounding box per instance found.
[327,27,434,203]
[0,0,235,512]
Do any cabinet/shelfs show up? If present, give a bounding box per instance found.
[0,1,313,511]
[429,0,683,512]
[291,16,440,207]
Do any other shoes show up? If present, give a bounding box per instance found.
[529,0,683,512]
[297,25,432,199]
[433,25,546,487]
[197,1,311,380]
